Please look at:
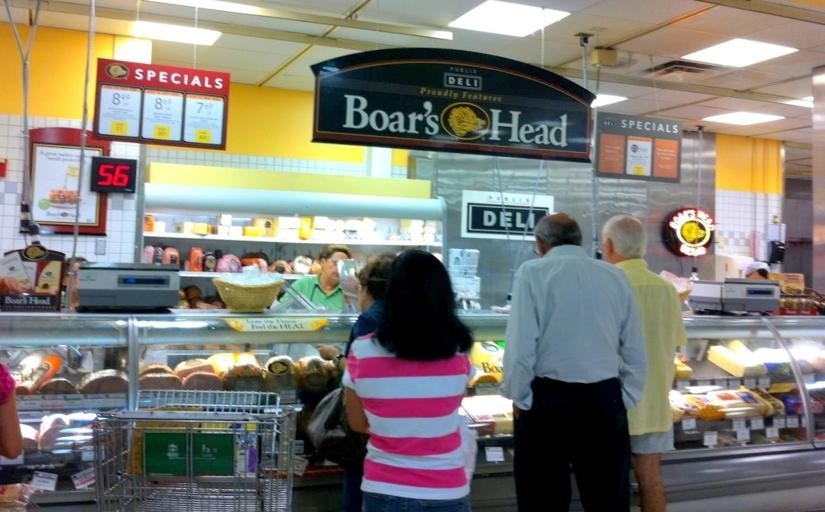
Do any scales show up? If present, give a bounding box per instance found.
[76,260,180,312]
[688,278,781,315]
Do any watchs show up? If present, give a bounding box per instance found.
[331,354,347,368]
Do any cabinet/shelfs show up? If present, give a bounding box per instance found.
[136,201,445,355]
[0,310,825,512]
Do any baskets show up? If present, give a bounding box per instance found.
[212,278,285,313]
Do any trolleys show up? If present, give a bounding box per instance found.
[93,390,295,512]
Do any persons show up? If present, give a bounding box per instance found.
[498,215,648,512]
[0,362,22,459]
[269,244,352,362]
[315,252,397,511]
[744,261,769,280]
[343,250,473,511]
[52,255,94,371]
[601,215,688,511]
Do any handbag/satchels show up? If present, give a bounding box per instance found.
[307,387,371,478]
